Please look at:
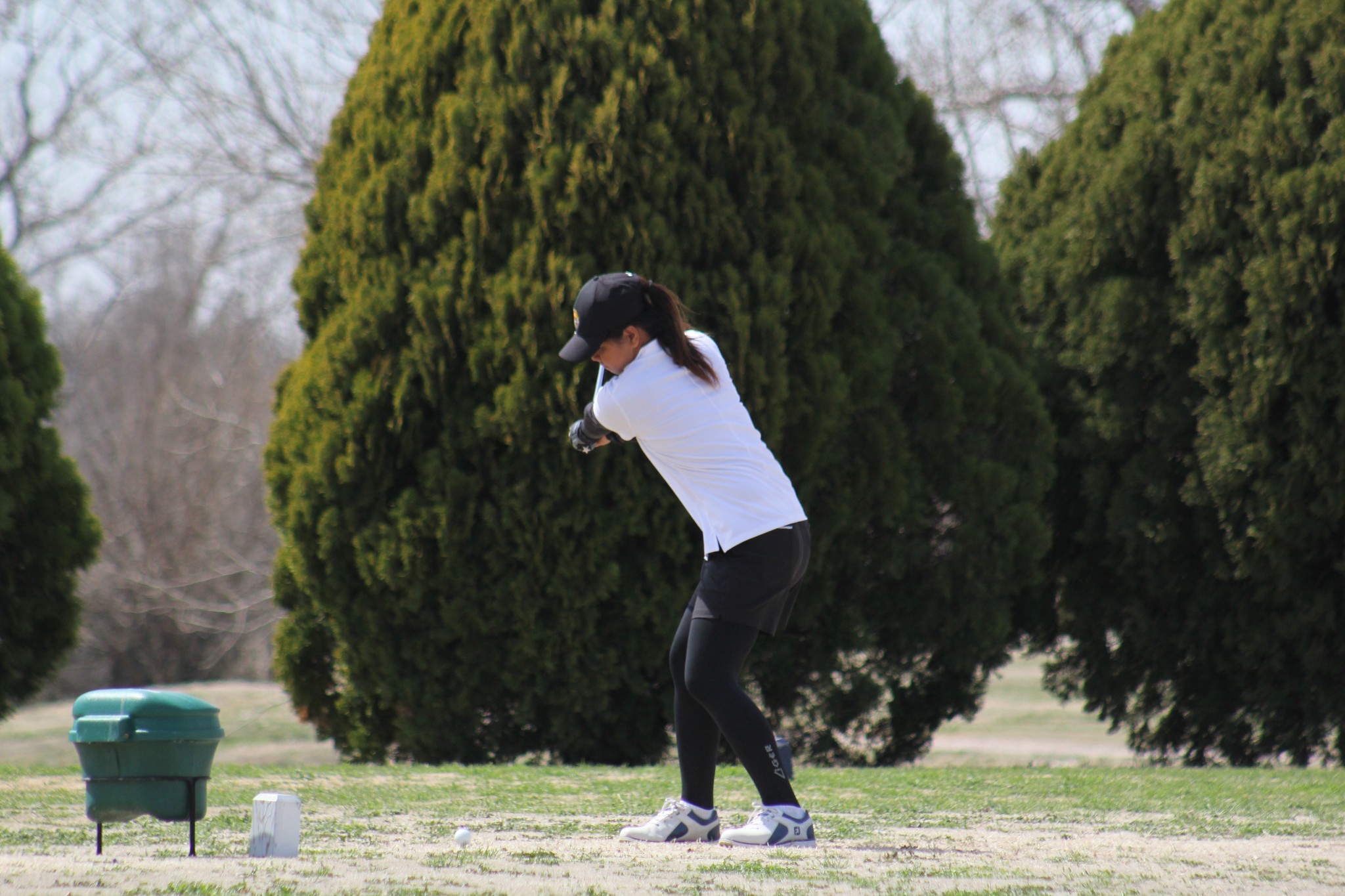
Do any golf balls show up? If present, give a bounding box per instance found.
[454,830,472,845]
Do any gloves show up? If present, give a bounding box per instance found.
[568,420,596,454]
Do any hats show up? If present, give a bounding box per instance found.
[558,272,653,363]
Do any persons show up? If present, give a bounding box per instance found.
[558,272,816,845]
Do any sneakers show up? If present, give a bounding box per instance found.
[619,798,721,843]
[718,802,817,848]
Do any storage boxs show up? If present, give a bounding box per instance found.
[252,792,300,857]
[67,690,222,822]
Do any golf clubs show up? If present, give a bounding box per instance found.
[581,364,607,452]
